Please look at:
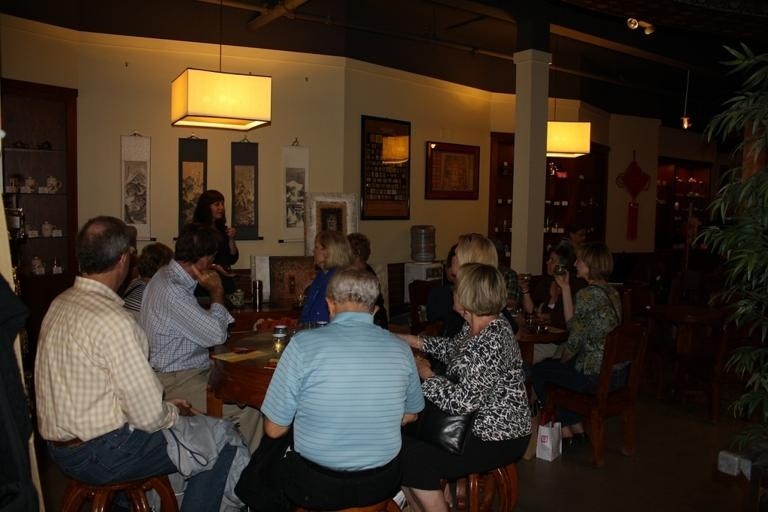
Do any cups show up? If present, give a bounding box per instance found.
[316,321,328,327]
[42,221,57,237]
[31,258,43,272]
[554,263,565,275]
[6,172,64,195]
[269,325,289,355]
[518,311,553,336]
[521,272,532,282]
[229,288,246,309]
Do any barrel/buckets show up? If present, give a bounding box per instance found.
[410,225,436,262]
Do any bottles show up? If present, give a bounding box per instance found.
[251,280,263,309]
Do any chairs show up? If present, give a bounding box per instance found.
[646,233,690,301]
[545,323,648,467]
[621,279,658,322]
[688,318,760,422]
[528,274,553,307]
[408,278,443,335]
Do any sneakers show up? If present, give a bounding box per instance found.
[561,432,591,452]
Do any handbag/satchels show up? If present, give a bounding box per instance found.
[535,412,563,462]
[402,353,480,459]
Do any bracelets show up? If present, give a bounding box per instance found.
[211,264,216,269]
[416,335,420,348]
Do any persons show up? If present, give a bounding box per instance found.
[387,264,531,511]
[140,220,266,458]
[190,188,240,298]
[449,232,519,338]
[29,215,237,512]
[259,263,426,511]
[295,229,355,325]
[423,220,592,337]
[119,242,172,313]
[347,232,388,331]
[527,237,624,458]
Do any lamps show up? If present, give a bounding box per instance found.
[380,135,410,164]
[171,0,272,133]
[546,64,592,158]
[680,69,692,130]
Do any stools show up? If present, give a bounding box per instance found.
[297,498,402,511]
[439,463,520,512]
[62,477,179,512]
[656,300,729,404]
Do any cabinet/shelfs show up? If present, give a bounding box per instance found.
[655,155,712,240]
[489,132,608,250]
[0,75,78,372]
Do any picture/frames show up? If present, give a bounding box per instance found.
[302,190,361,257]
[361,113,411,219]
[424,140,481,201]
